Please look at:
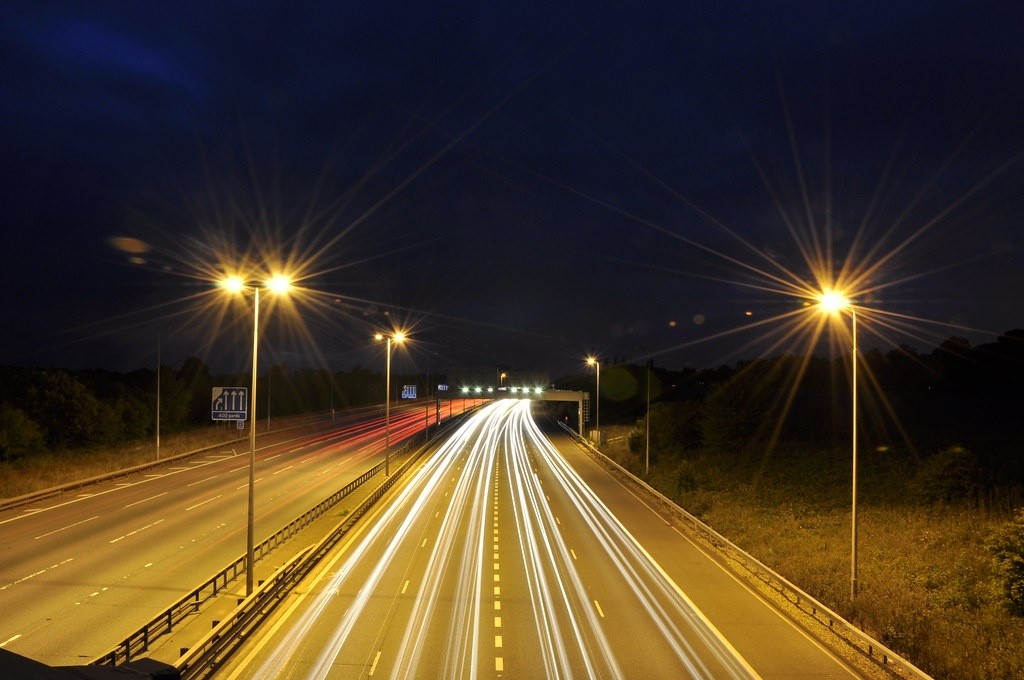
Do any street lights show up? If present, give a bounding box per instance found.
[219,262,294,597]
[586,355,598,452]
[816,290,859,602]
[376,325,406,478]
